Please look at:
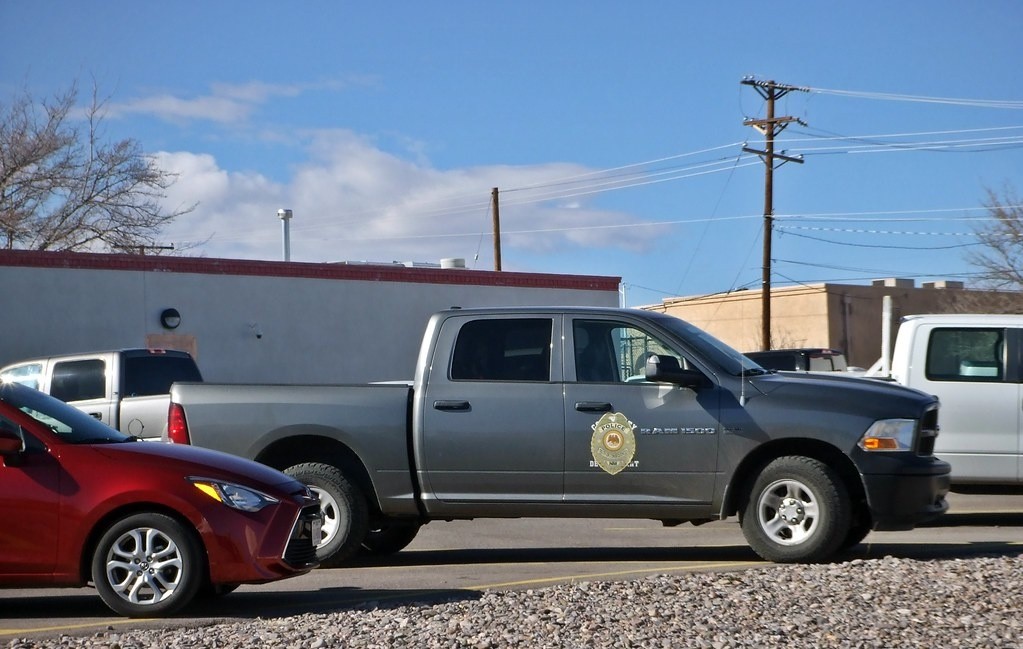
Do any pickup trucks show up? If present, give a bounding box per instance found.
[777,314,1023,484]
[167,306,951,562]
[0,349,203,442]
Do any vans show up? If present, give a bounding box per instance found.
[742,348,847,373]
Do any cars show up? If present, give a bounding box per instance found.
[0,379,320,619]
